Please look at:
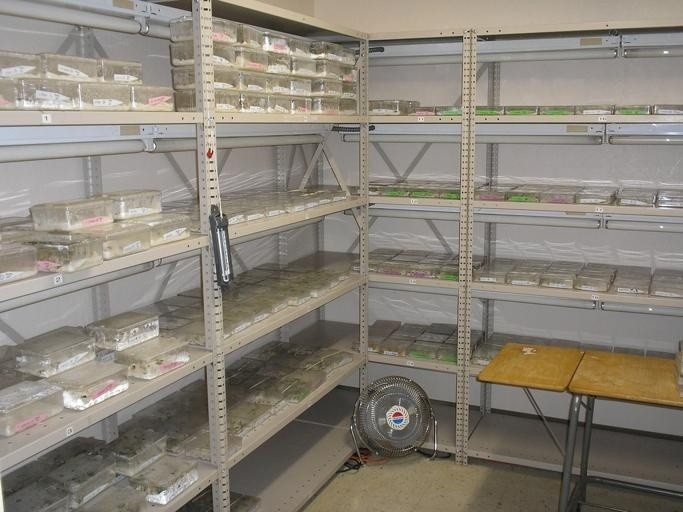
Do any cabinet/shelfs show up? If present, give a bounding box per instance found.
[0,0,361,512]
[362,24,683,501]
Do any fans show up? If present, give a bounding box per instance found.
[349,374,440,466]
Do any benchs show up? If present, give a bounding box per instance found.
[476,341,681,512]
